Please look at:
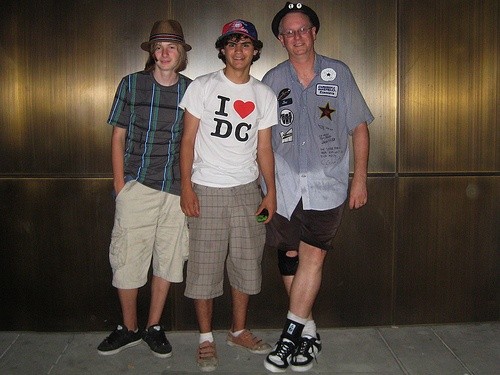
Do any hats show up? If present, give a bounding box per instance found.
[271,2,320,39]
[140,20,192,53]
[215,20,259,49]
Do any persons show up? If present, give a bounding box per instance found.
[177,20,278,371]
[96,20,196,354]
[263,2,373,372]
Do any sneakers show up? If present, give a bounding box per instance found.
[263,333,297,372]
[227,329,273,354]
[196,339,217,372]
[141,324,173,358]
[97,321,142,355]
[290,333,321,371]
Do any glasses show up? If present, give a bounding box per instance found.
[279,25,314,37]
